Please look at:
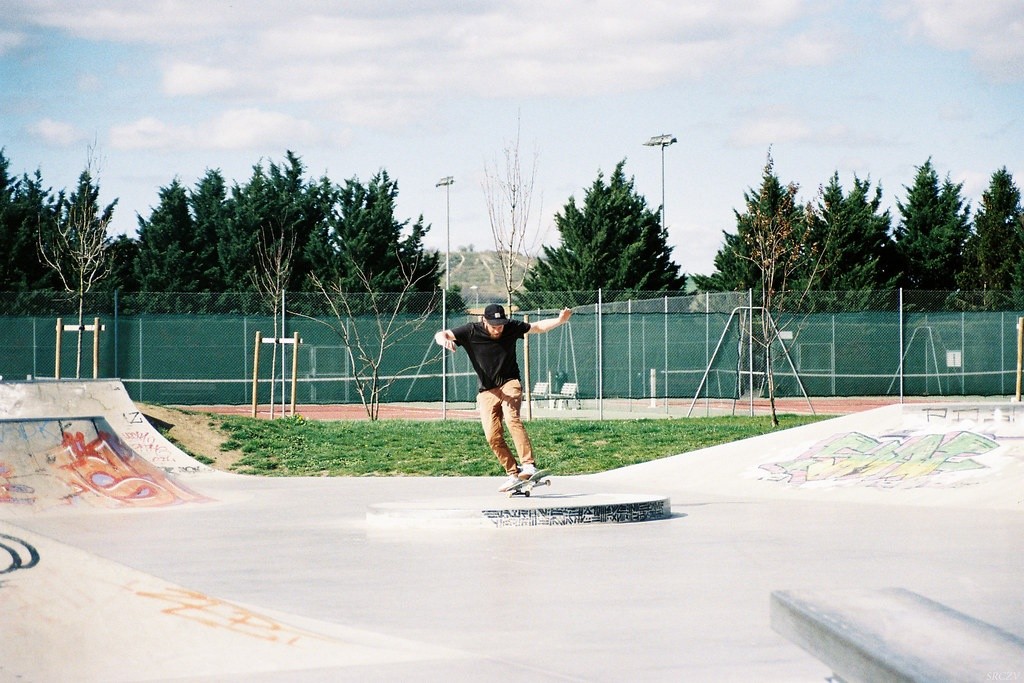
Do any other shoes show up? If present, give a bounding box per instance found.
[517,464,538,481]
[498,477,527,492]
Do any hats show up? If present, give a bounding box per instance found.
[484,304,509,325]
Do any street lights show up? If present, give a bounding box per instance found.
[434,175,457,291]
[643,133,678,234]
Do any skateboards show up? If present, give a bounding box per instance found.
[504,468,552,498]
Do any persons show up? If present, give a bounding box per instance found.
[435,304,572,491]
